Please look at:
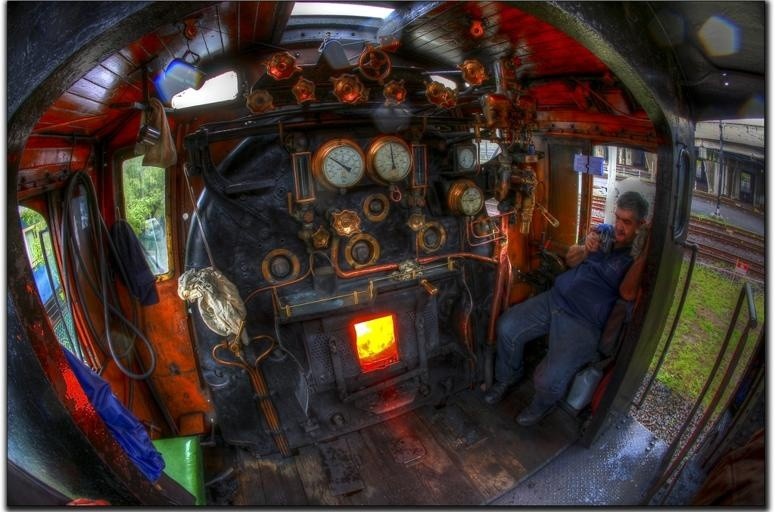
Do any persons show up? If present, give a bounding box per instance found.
[482,191,653,428]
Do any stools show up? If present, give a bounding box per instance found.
[152,436,212,507]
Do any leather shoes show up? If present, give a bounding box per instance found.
[483,381,514,405]
[515,401,556,427]
